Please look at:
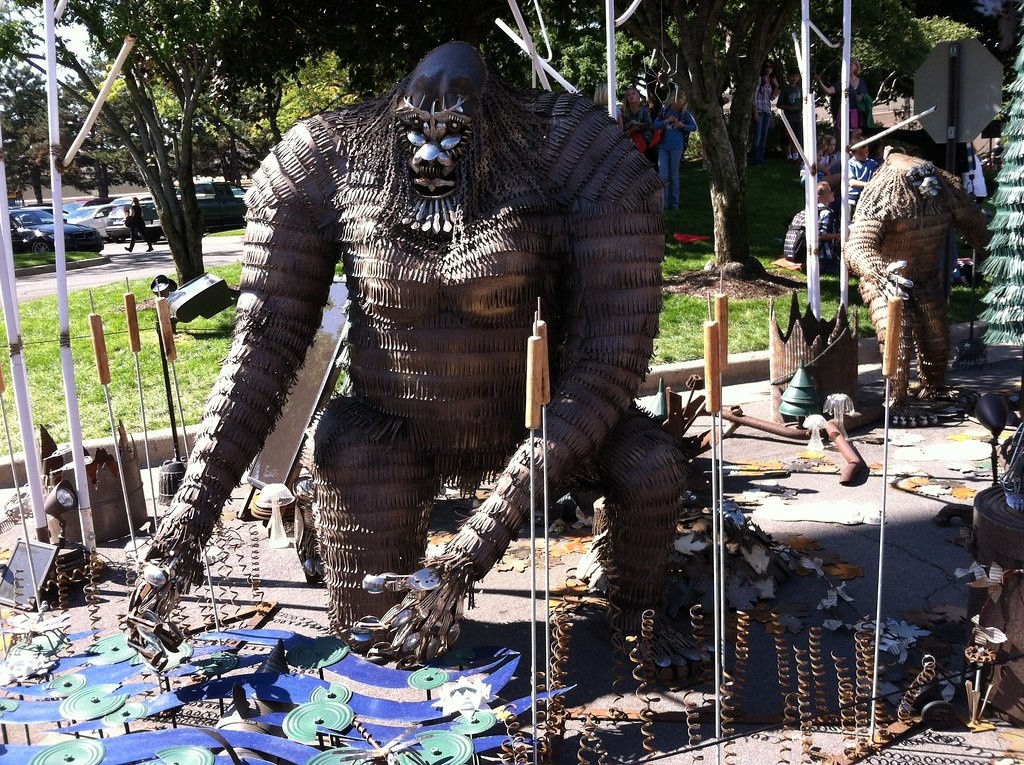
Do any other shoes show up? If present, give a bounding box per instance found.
[670,205,680,212]
[954,276,971,288]
[125,247,132,252]
[793,157,799,163]
[785,158,793,161]
[147,248,153,252]
[750,159,766,167]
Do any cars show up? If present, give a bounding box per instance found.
[26,197,143,244]
[8,208,105,254]
[230,186,247,198]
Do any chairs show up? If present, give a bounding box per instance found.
[24,216,31,226]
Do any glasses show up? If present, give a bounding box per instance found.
[765,65,772,68]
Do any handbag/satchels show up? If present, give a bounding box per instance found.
[837,108,858,131]
[125,216,136,227]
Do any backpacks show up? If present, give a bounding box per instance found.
[784,207,834,261]
[664,106,689,152]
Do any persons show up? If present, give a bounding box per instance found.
[962,144,994,219]
[751,58,779,166]
[713,51,729,121]
[784,132,961,279]
[130,41,719,693]
[844,148,994,426]
[595,84,623,132]
[621,88,652,160]
[650,88,697,211]
[814,58,868,150]
[987,140,1006,174]
[776,69,802,162]
[124,197,154,253]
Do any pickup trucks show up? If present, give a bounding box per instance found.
[141,183,248,243]
